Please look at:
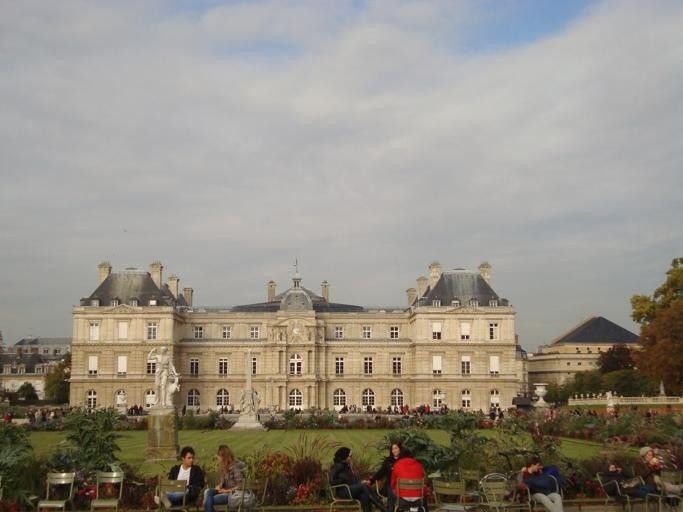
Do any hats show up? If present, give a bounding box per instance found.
[334,447,350,459]
[640,447,656,463]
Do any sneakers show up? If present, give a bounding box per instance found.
[154,496,160,504]
[161,496,171,508]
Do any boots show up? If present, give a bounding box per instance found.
[364,493,389,512]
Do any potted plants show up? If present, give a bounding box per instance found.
[0,421,35,512]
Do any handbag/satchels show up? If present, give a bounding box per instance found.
[228,490,255,507]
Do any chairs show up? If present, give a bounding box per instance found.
[38,467,682,512]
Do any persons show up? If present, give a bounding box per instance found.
[502,463,560,503]
[496,407,504,419]
[3,404,143,425]
[147,346,180,409]
[516,455,563,511]
[328,446,385,511]
[489,405,496,420]
[201,445,244,512]
[633,446,683,497]
[180,403,449,416]
[389,449,428,512]
[601,463,681,507]
[153,445,205,509]
[369,441,403,511]
